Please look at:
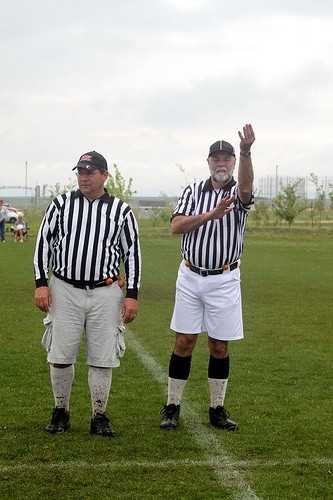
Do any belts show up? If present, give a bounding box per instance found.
[181,256,240,279]
[51,270,120,292]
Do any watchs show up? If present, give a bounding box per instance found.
[240,151,251,157]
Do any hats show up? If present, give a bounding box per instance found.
[71,150,109,174]
[208,139,235,160]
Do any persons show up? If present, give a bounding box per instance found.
[13,216,24,242]
[159,124,255,430]
[33,150,141,437]
[0,199,6,242]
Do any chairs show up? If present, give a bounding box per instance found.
[10,222,30,242]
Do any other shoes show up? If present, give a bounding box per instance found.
[3,240,6,242]
[13,239,24,243]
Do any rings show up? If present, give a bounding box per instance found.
[134,314,137,317]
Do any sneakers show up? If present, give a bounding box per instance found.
[206,407,238,431]
[40,408,72,438]
[85,415,119,437]
[158,405,184,430]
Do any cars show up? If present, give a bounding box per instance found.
[3,204,18,224]
